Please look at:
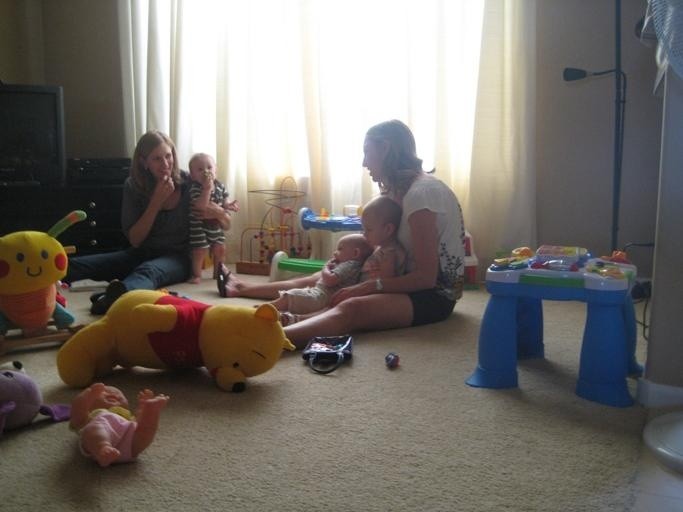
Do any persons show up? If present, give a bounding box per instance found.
[90,131,233,315]
[69,381,170,468]
[270,234,372,314]
[279,196,408,327]
[216,120,464,353]
[187,154,240,284]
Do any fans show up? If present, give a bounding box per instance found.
[644,0,683,473]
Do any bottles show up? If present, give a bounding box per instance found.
[344,205,362,218]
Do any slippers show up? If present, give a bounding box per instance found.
[217,261,232,297]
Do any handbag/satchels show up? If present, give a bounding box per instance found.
[303,334,353,373]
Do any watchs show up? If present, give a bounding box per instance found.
[376,279,383,294]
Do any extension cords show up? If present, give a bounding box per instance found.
[631,278,653,303]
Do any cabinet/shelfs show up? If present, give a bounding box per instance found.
[0,184,131,258]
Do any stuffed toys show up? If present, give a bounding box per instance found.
[0,210,87,337]
[0,360,73,436]
[56,289,297,392]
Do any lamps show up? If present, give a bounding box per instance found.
[563,0,654,255]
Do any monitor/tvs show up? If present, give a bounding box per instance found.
[0,83,66,187]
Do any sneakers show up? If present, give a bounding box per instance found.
[89,279,127,315]
[281,311,298,326]
